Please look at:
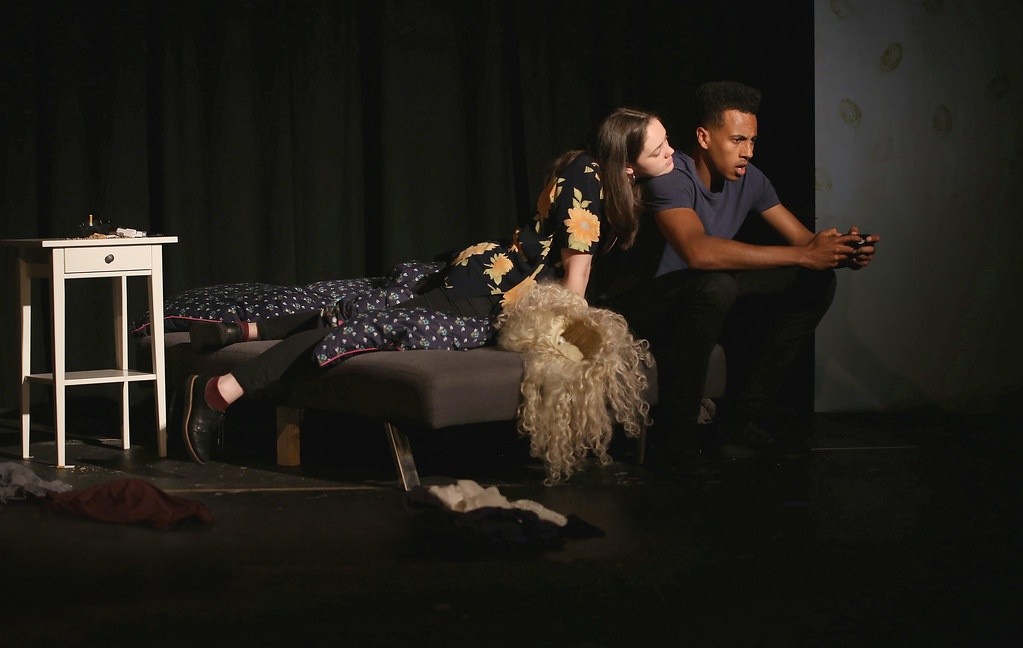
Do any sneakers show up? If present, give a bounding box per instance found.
[181,374,227,466]
[189,319,243,356]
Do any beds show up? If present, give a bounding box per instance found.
[134,284,730,495]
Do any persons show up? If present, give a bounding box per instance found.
[590,81,881,478]
[182,109,677,464]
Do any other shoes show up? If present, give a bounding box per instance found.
[717,414,783,450]
[656,423,703,475]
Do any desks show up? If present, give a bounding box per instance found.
[0,235,181,464]
